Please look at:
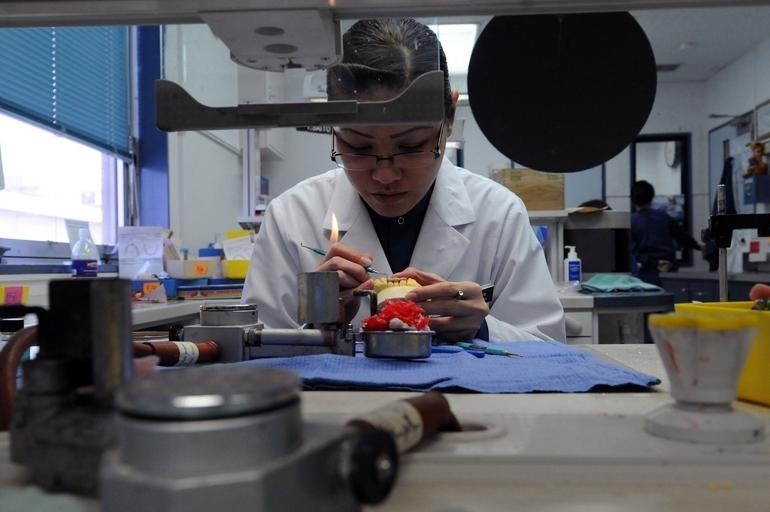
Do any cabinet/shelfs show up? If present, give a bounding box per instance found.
[663,272,770,302]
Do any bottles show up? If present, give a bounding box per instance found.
[72,228,99,279]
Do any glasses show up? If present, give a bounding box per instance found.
[329,115,449,173]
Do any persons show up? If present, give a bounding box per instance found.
[242,16,567,343]
[631,179,705,270]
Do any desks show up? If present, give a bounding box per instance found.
[127,297,246,335]
[2,341,767,511]
